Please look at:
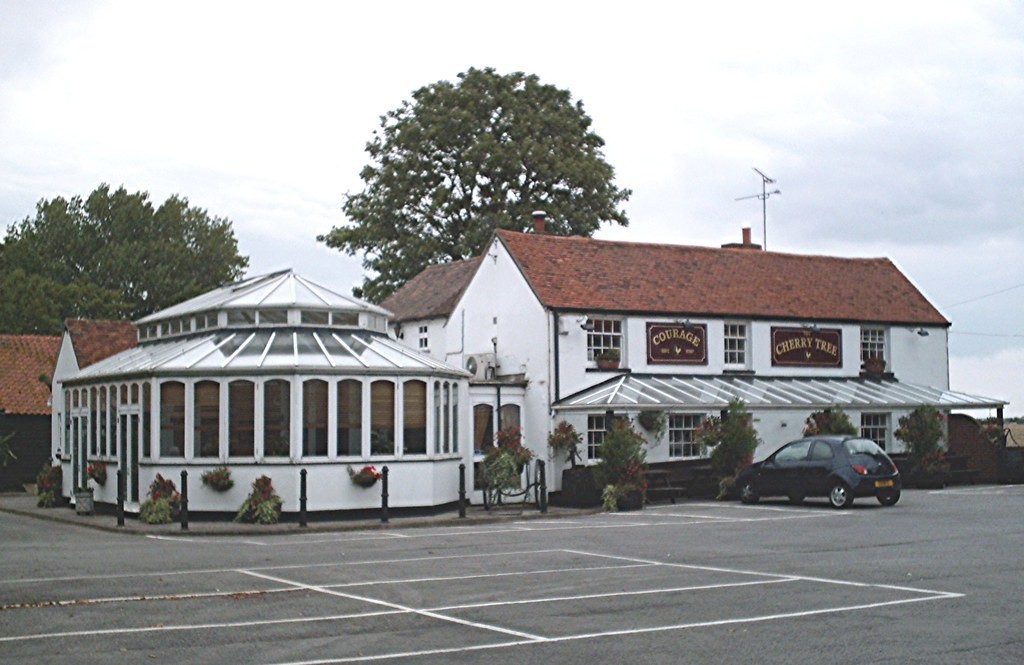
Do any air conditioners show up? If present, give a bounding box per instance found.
[461,352,495,379]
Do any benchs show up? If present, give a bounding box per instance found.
[644,480,688,493]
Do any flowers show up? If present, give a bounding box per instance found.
[200,464,234,491]
[547,419,585,462]
[138,472,188,524]
[232,476,284,523]
[635,410,668,449]
[598,419,649,490]
[483,426,536,467]
[87,463,108,487]
[38,458,57,497]
[893,401,948,471]
[347,465,382,488]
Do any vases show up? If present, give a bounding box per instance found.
[618,490,642,513]
[915,471,945,490]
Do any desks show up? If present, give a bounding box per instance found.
[642,470,677,500]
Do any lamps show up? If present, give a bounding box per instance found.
[801,320,822,333]
[675,315,696,328]
[576,312,595,330]
[905,326,928,336]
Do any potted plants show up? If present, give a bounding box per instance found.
[596,348,622,368]
[861,354,887,372]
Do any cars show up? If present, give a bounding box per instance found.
[731,433,901,510]
